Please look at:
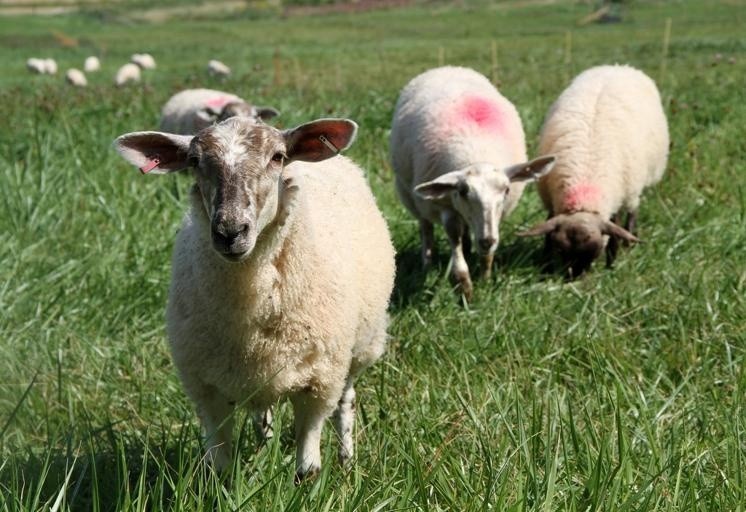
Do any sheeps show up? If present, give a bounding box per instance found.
[388,62,559,302]
[512,61,672,284]
[112,112,399,512]
[159,88,279,135]
[24,50,157,87]
[207,60,231,83]
